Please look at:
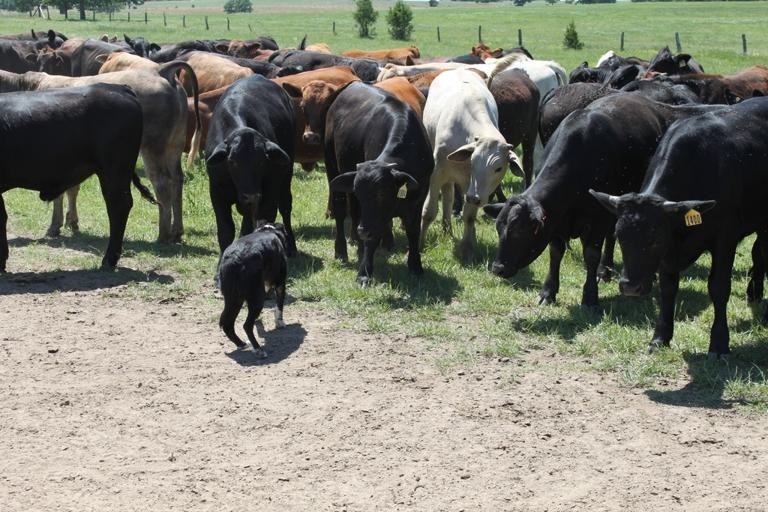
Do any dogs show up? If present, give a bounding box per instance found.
[218,218,289,359]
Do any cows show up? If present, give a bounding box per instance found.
[422,68,525,270]
[482,85,703,308]
[1,59,200,246]
[0,82,164,273]
[314,78,435,286]
[281,76,427,219]
[1,25,768,94]
[488,59,568,201]
[589,95,763,358]
[202,74,299,261]
[197,65,361,173]
